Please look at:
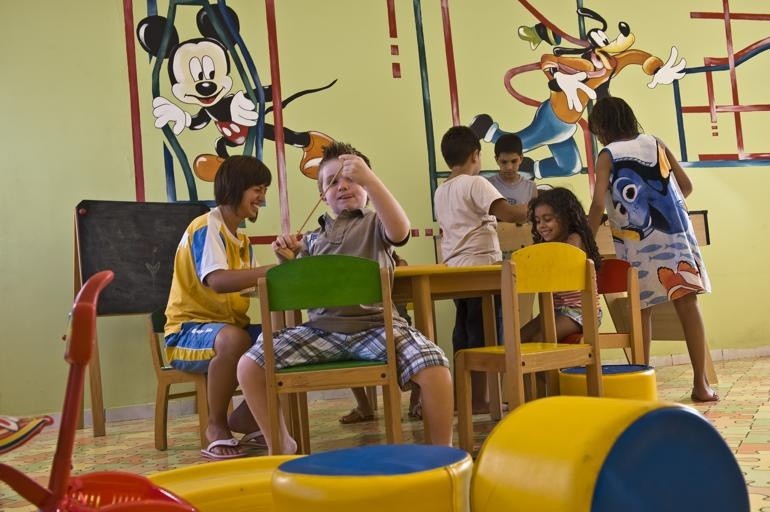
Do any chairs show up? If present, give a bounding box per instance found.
[455,243,603,453]
[549,258,646,371]
[258,255,401,458]
[149,305,242,450]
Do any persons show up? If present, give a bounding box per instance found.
[237,141,454,457]
[434,126,531,414]
[487,134,538,206]
[587,97,720,402]
[339,251,423,424]
[163,155,278,459]
[519,187,603,402]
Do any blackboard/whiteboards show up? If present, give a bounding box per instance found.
[75,199,211,316]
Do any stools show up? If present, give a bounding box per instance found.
[559,363,659,406]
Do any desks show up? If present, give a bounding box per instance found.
[243,263,561,456]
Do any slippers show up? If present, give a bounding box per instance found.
[406,400,425,420]
[199,436,248,461]
[338,406,376,425]
[238,429,270,450]
